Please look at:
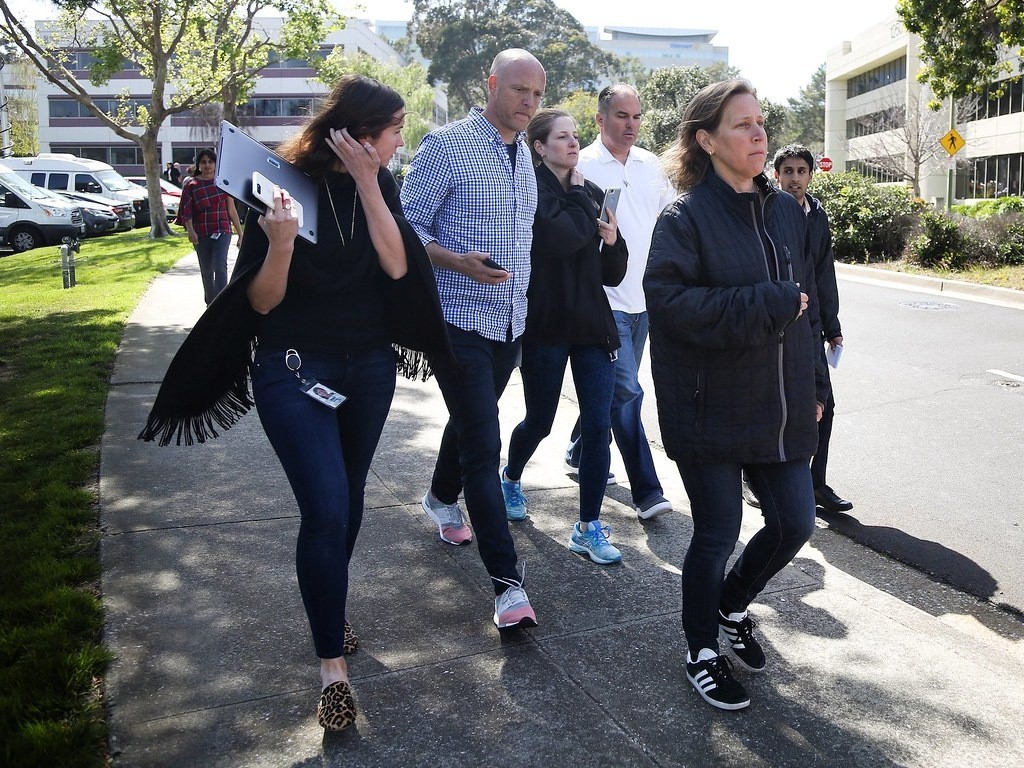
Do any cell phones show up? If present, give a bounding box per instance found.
[252,172,302,227]
[483,258,509,273]
[598,188,622,226]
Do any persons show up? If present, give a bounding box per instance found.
[499,108,629,562]
[313,388,334,399]
[163,162,195,189]
[137,70,457,729]
[643,83,828,712]
[563,83,676,519]
[742,143,853,512]
[173,149,243,306]
[396,47,546,630]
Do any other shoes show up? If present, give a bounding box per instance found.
[344,620,360,654]
[317,680,356,732]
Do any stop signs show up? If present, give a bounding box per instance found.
[819,158,833,171]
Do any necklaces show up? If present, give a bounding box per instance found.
[323,174,357,247]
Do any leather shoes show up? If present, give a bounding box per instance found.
[813,487,853,511]
[741,481,760,508]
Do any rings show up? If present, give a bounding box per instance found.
[284,204,292,209]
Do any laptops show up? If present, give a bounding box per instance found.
[213,119,319,247]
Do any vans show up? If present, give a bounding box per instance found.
[0,164,86,254]
[0,153,150,229]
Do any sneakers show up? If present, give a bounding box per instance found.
[499,465,527,519]
[492,586,538,630]
[422,486,473,546]
[569,520,623,564]
[718,607,766,671]
[686,647,751,710]
[636,493,673,519]
[563,458,616,484]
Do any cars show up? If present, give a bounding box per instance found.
[34,184,135,239]
[124,179,183,220]
